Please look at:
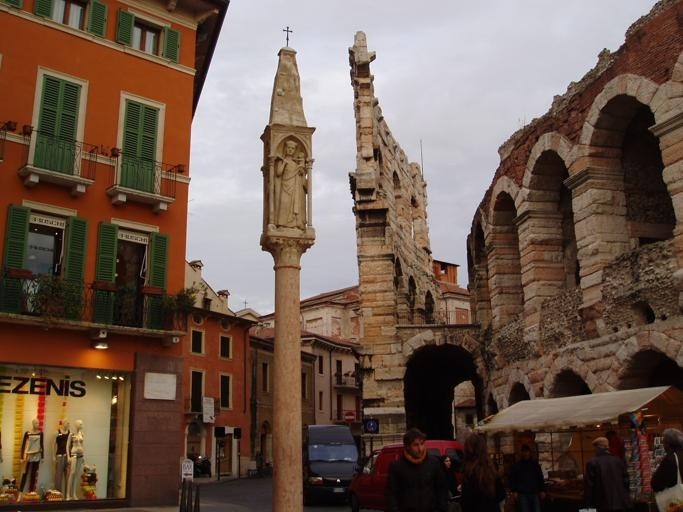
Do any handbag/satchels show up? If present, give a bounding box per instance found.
[655,484,683,512]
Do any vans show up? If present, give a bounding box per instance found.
[300,421,363,501]
[346,439,472,511]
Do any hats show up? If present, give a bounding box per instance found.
[591,437,610,447]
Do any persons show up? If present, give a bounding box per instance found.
[383,428,547,512]
[650,428,683,512]
[53,418,72,491]
[16,418,45,502]
[582,436,626,512]
[65,419,85,501]
[274,140,307,230]
